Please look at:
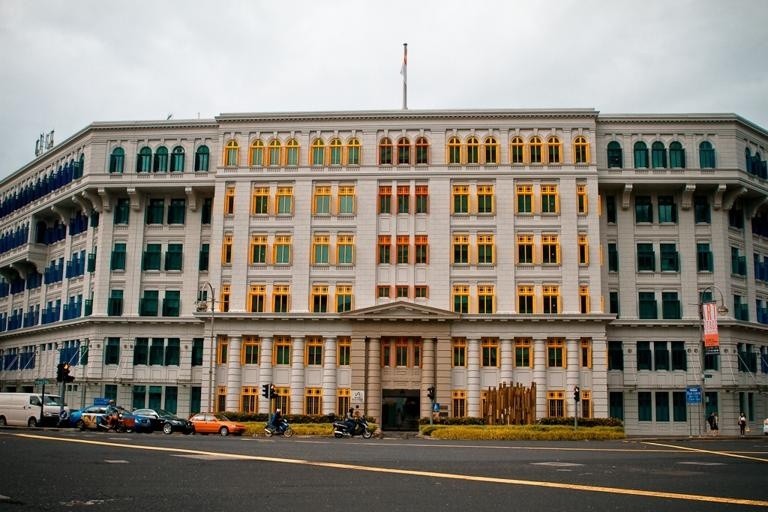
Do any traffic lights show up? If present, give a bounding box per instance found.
[428,387,435,400]
[262,384,276,399]
[574,388,579,402]
[57,363,69,383]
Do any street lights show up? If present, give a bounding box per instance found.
[199,281,215,412]
[700,284,729,437]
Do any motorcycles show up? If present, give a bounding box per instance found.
[265,419,292,438]
[333,418,373,439]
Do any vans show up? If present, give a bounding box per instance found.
[0,392,68,427]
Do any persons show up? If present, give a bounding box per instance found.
[706,411,719,436]
[105,398,115,417]
[738,411,746,438]
[344,407,358,435]
[270,407,281,432]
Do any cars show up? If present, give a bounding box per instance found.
[70,405,247,436]
